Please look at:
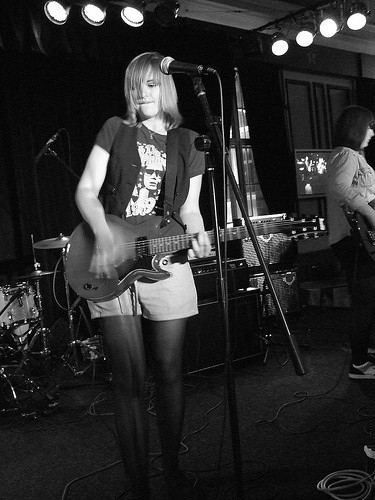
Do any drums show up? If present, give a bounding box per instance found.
[0,280,41,329]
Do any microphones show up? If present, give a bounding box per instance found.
[160,56,216,75]
[34,128,63,164]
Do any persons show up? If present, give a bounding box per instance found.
[74,53,212,500]
[326,107,375,379]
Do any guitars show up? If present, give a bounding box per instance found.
[354,198,375,262]
[63,213,331,304]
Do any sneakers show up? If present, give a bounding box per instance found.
[349,360,375,379]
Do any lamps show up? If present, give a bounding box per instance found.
[43,0,73,25]
[154,0,181,26]
[295,22,317,48]
[120,0,147,27]
[346,2,368,31]
[81,0,108,26]
[270,32,289,56]
[318,10,343,38]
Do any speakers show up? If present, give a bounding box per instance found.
[182,286,264,378]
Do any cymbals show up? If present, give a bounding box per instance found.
[13,269,61,279]
[32,235,71,250]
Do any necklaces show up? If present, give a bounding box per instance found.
[147,127,159,140]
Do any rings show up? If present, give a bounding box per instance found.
[95,250,100,252]
